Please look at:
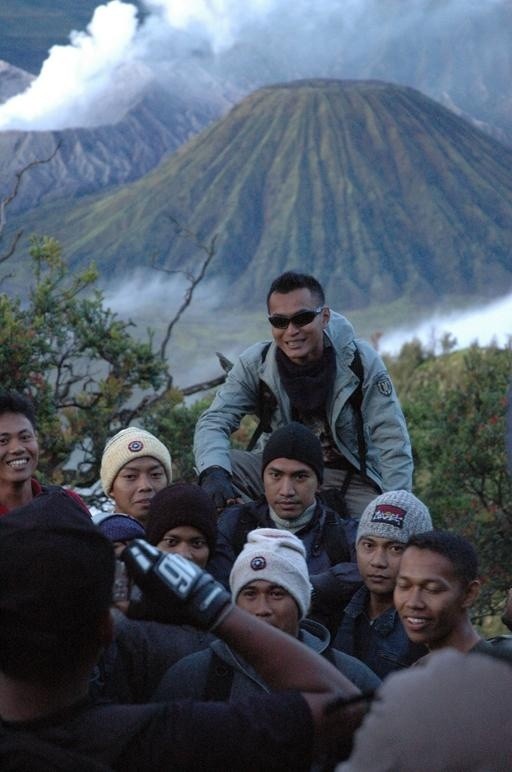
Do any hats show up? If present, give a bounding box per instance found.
[89,513,146,555]
[260,424,325,487]
[100,426,173,499]
[1,484,114,639]
[355,489,434,550]
[148,484,219,558]
[224,528,314,622]
[334,646,512,771]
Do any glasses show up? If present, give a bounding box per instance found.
[266,307,326,327]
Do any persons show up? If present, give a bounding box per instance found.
[189,271,415,523]
[1,389,511,771]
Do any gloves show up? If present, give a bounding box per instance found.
[110,538,230,631]
